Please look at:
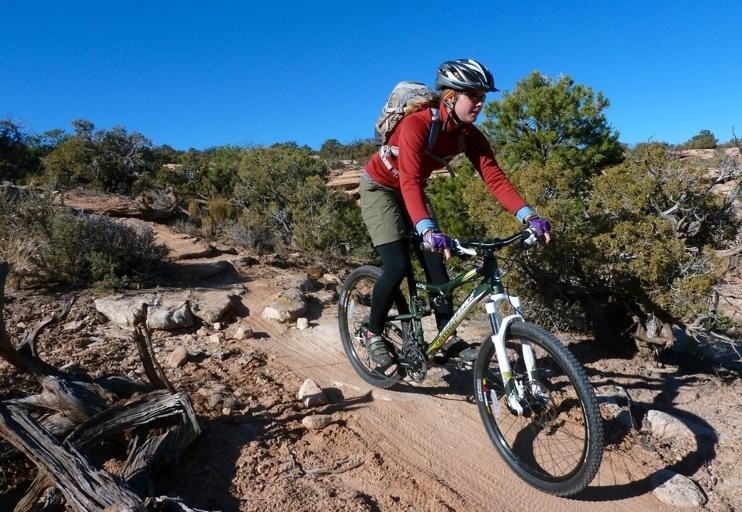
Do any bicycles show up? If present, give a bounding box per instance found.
[338,223,605,497]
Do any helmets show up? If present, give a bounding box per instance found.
[435,58,500,92]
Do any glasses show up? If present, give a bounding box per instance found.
[463,93,486,103]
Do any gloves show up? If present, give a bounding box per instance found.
[421,228,453,250]
[527,213,551,236]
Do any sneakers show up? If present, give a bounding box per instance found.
[441,333,481,361]
[363,329,392,366]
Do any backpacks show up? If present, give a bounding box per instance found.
[374,80,440,157]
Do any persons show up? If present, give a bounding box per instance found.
[358,57,552,370]
